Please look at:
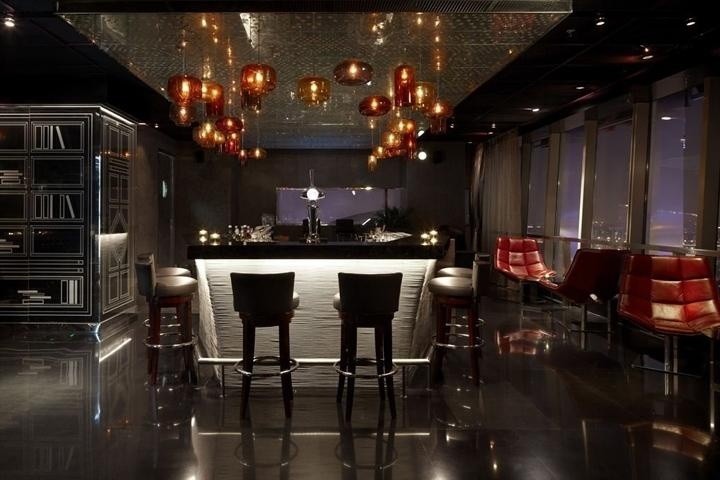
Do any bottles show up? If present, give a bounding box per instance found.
[225,224,253,246]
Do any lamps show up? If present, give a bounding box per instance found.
[298,185,326,243]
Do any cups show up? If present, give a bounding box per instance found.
[199,230,208,246]
[420,234,430,246]
[209,234,221,246]
[429,230,439,246]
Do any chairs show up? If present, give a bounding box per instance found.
[232,403,298,480]
[333,271,402,402]
[428,252,492,386]
[140,387,199,480]
[336,219,353,240]
[334,404,398,480]
[230,272,298,401]
[136,252,195,381]
[303,219,321,242]
